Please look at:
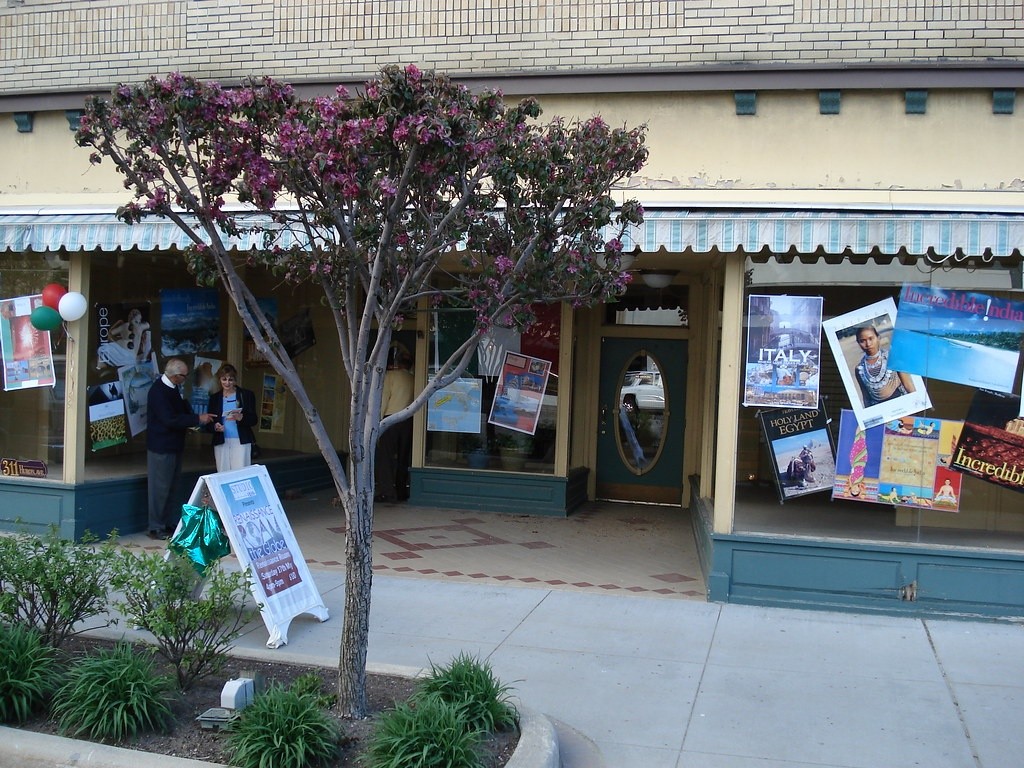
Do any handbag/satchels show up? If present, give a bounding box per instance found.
[181,398,195,416]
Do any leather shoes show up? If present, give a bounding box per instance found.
[150,529,172,540]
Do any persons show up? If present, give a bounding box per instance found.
[97,308,150,369]
[146,358,218,540]
[375,353,415,503]
[854,324,916,409]
[787,445,816,481]
[205,364,257,472]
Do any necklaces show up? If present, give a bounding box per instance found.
[865,350,880,359]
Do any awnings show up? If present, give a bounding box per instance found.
[1,205,1024,270]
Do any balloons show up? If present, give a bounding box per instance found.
[42,284,67,313]
[29,305,62,331]
[57,291,87,322]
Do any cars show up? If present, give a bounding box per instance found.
[620,373,666,416]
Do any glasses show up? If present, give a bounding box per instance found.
[176,373,190,380]
